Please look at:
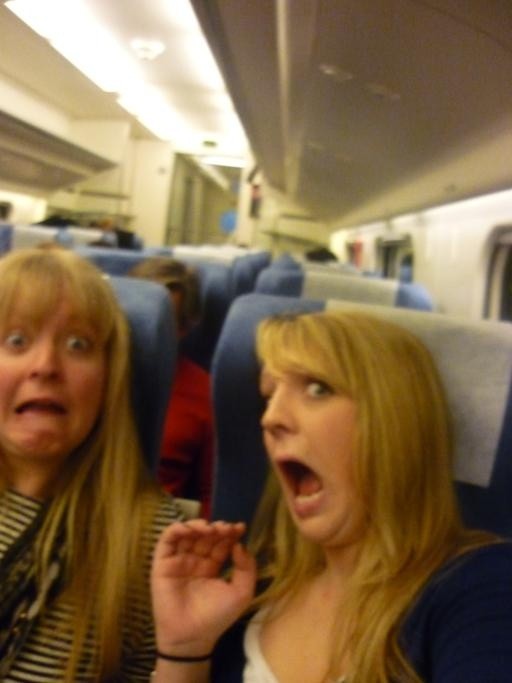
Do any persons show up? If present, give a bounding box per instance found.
[148,306,510,683]
[129,254,219,525]
[1,247,189,682]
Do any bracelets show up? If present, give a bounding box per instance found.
[156,650,214,663]
